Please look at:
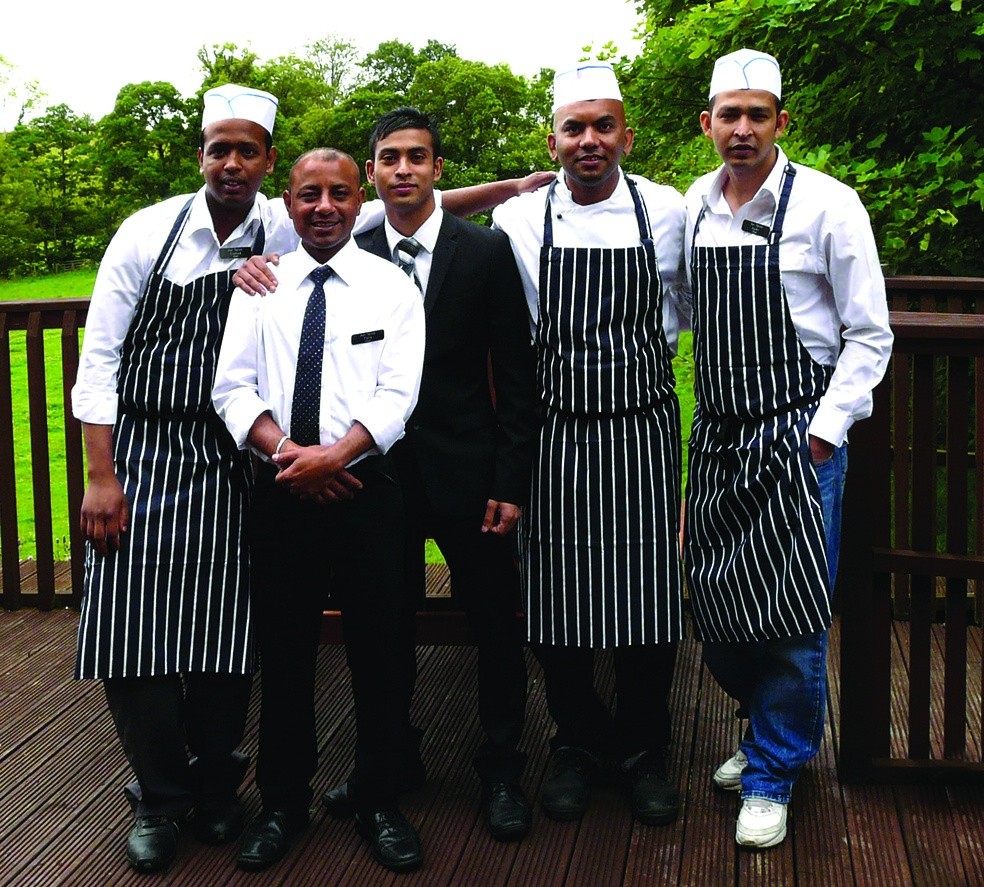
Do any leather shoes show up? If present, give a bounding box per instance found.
[541,742,599,822]
[236,807,310,873]
[354,805,424,871]
[125,815,180,871]
[323,757,426,814]
[482,775,533,841]
[613,747,681,829]
[192,801,241,843]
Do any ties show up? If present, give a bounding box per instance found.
[291,266,333,449]
[396,240,424,296]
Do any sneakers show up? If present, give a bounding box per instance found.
[735,796,789,849]
[713,748,750,791]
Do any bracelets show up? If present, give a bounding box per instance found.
[273,434,290,471]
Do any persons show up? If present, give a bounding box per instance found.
[212,147,427,870]
[685,50,895,847]
[357,109,529,870]
[68,81,558,870]
[492,60,686,822]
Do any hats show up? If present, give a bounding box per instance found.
[201,83,280,136]
[553,60,624,116]
[709,48,782,103]
